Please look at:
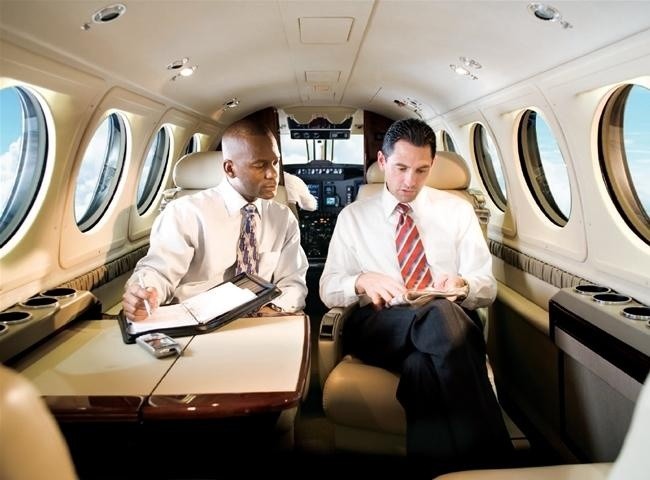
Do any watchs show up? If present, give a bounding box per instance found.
[267,303,282,313]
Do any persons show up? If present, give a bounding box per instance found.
[283,172,318,213]
[119,120,310,323]
[318,118,521,480]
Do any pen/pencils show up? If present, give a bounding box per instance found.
[138,271,154,317]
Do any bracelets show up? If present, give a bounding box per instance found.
[455,278,471,302]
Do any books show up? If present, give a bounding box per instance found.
[384,285,469,306]
[129,281,257,334]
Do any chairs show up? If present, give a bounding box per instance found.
[157,150,312,451]
[317,150,500,457]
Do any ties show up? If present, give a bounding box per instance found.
[233,205,260,278]
[393,204,435,291]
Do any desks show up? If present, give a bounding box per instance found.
[6,311,313,421]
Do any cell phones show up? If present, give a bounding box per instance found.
[136,331,180,357]
